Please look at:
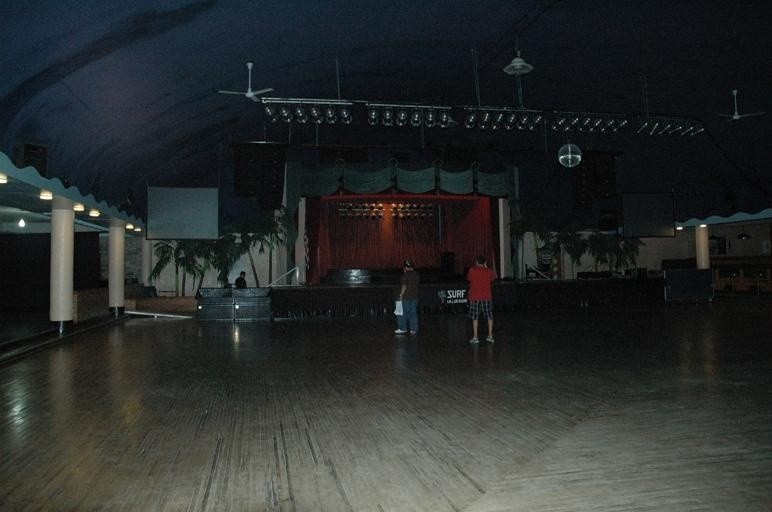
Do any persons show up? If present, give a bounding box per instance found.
[235,271,247,288]
[466,255,497,344]
[394,259,420,334]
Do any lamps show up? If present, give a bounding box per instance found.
[40,189,52,200]
[338,202,385,219]
[463,105,544,131]
[125,223,135,230]
[133,226,142,232]
[261,97,354,124]
[0,172,8,184]
[635,113,704,136]
[73,202,84,211]
[552,110,630,133]
[365,103,453,129]
[390,204,434,219]
[88,208,101,217]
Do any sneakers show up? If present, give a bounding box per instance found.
[487,336,493,342]
[470,338,481,344]
[394,328,417,333]
